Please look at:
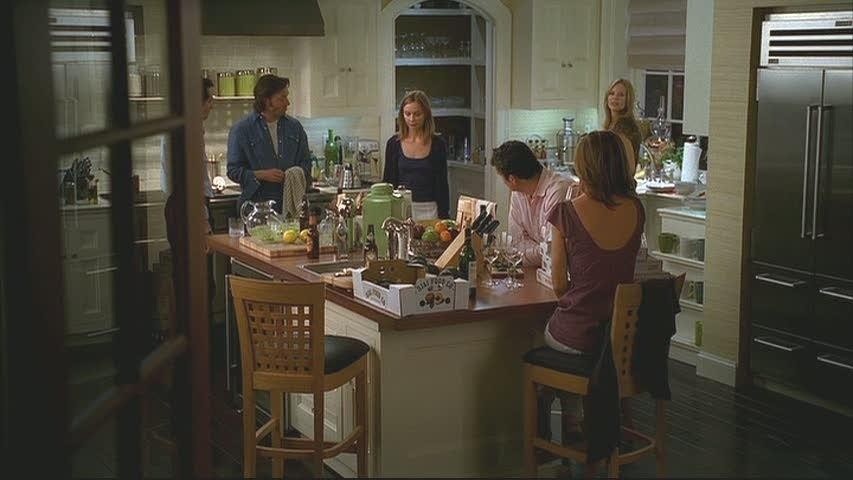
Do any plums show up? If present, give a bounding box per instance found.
[412,226,423,238]
[450,229,457,239]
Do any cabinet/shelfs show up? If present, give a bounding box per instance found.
[60,208,114,347]
[394,14,489,172]
[651,209,706,366]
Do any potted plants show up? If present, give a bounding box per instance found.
[659,140,685,184]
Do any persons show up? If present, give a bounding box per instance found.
[227,74,311,224]
[546,131,647,443]
[603,78,640,179]
[163,78,216,311]
[490,141,575,267]
[383,91,449,220]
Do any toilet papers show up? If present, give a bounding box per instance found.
[680,141,703,184]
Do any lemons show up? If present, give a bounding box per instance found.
[282,230,297,243]
[299,229,307,242]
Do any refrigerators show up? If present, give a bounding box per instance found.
[744,12,851,419]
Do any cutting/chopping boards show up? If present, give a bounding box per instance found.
[236,232,358,259]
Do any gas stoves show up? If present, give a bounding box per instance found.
[101,188,243,202]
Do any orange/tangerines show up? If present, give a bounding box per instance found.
[421,231,438,240]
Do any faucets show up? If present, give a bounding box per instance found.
[640,142,656,169]
[381,215,415,261]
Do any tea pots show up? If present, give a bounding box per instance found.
[239,198,283,238]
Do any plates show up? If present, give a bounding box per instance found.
[646,180,675,194]
[685,193,705,213]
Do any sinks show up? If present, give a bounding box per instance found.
[297,259,365,273]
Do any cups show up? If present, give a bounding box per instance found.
[206,161,220,182]
[694,320,703,345]
[658,231,704,262]
[679,279,704,305]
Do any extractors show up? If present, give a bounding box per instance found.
[190,0,325,36]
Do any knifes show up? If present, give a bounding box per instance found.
[469,212,500,239]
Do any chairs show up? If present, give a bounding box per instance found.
[520,273,686,479]
[225,274,370,480]
[453,196,495,233]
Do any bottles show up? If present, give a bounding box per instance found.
[362,224,378,264]
[298,193,308,232]
[305,210,321,259]
[456,228,476,293]
[323,128,338,178]
[553,116,578,163]
[333,209,350,262]
[128,67,279,100]
